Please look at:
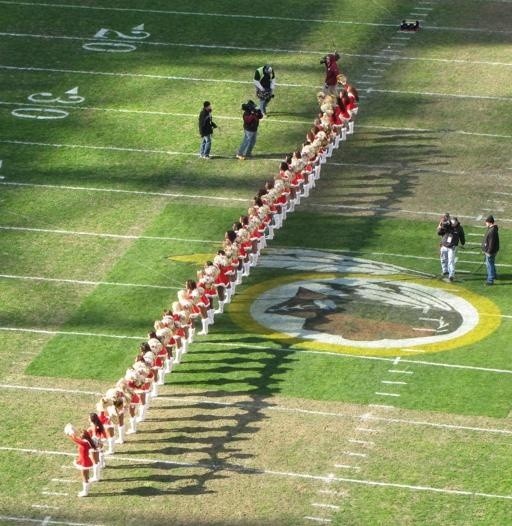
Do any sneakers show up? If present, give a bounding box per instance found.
[441,272,454,281]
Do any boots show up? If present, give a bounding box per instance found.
[112,392,149,444]
[77,438,115,497]
[326,122,354,158]
[151,328,195,397]
[235,236,268,285]
[264,191,301,240]
[196,281,236,335]
[300,154,327,198]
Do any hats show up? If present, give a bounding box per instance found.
[486,215,494,224]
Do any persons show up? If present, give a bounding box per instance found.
[436,212,467,280]
[481,215,500,284]
[199,101,214,159]
[236,101,263,160]
[64,276,211,498]
[278,53,360,212]
[195,175,288,325]
[253,66,275,118]
